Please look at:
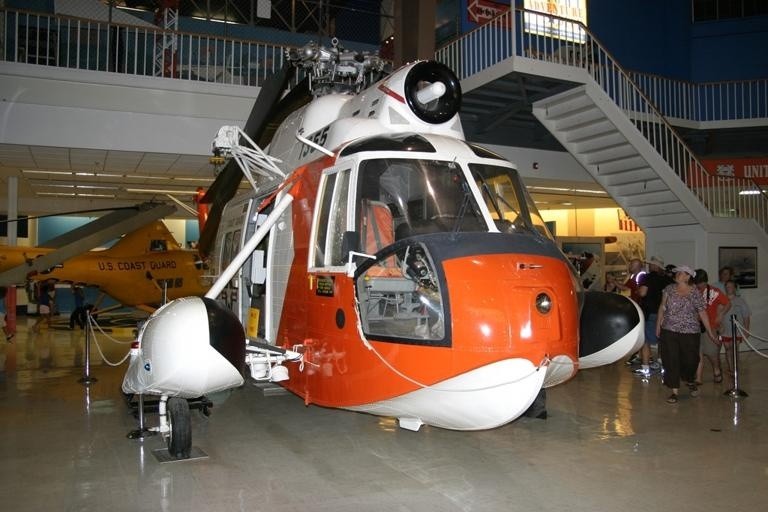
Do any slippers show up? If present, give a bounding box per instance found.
[729,368,735,379]
[713,368,723,383]
[686,381,704,385]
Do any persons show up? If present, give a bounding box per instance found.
[603,257,750,387]
[656,263,722,405]
[31,283,55,332]
[0,329,83,374]
[0,285,14,342]
[66,280,86,331]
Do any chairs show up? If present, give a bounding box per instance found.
[17,24,56,66]
[343,199,420,321]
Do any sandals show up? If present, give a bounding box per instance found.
[691,385,699,398]
[667,393,678,404]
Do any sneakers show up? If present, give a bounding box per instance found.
[627,353,662,376]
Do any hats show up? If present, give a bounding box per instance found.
[665,264,676,272]
[671,265,696,279]
[643,255,666,272]
